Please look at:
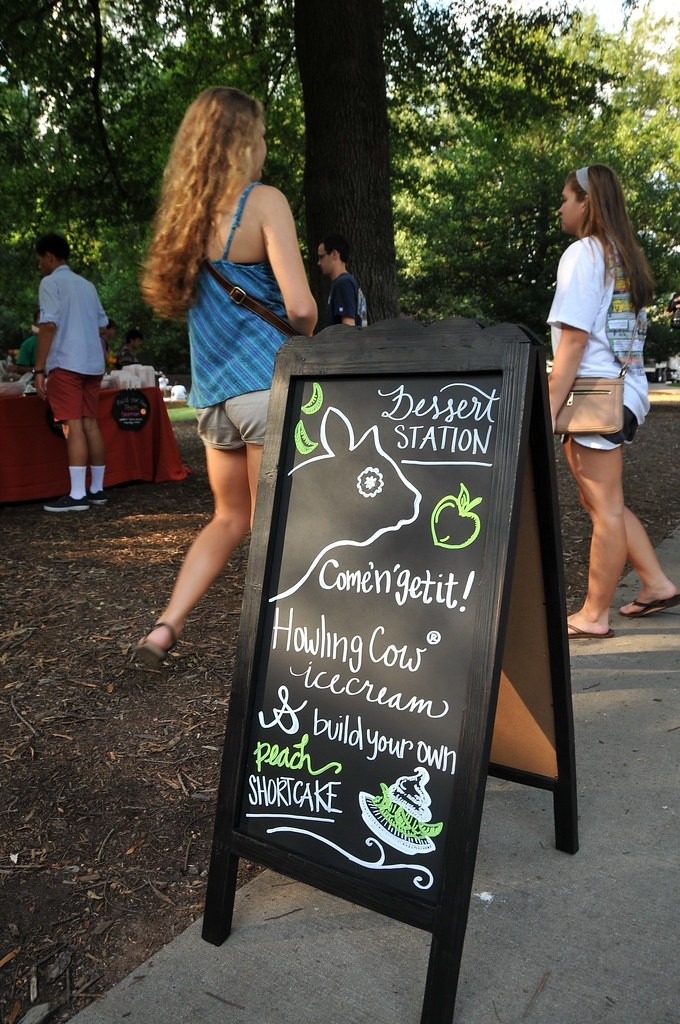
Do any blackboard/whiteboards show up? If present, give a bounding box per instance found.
[214,326,581,922]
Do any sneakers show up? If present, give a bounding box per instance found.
[86,491,108,505]
[43,495,90,512]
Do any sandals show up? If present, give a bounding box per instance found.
[134,623,177,672]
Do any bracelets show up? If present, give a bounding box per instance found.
[35,368,45,375]
[32,368,35,373]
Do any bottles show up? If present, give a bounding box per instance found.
[20,320,39,335]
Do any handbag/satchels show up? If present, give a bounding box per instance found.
[553,377,625,434]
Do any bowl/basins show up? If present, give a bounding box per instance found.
[99,376,112,389]
[0,382,27,397]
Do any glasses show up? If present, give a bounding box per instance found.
[319,250,332,261]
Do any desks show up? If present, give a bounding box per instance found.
[0,385,192,502]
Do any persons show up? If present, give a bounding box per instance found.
[133,87,318,669]
[316,235,367,330]
[100,319,116,363]
[16,310,47,378]
[546,164,680,640]
[34,233,109,512]
[159,374,186,400]
[117,328,139,370]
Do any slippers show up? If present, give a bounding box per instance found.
[619,593,680,618]
[568,623,616,640]
